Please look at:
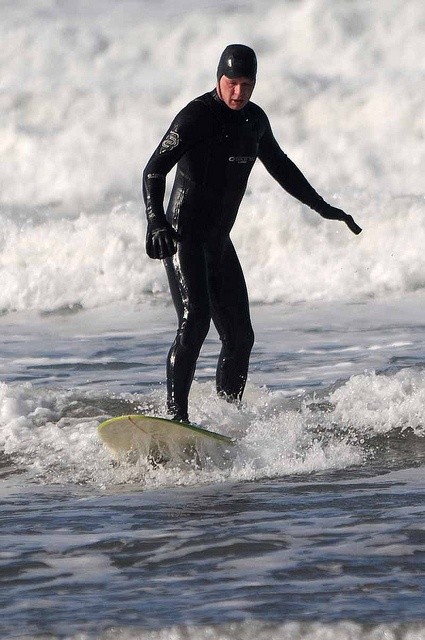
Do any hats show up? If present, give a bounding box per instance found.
[217,44,256,83]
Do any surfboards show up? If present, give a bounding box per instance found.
[98,414,238,456]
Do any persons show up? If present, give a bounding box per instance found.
[140,44,364,425]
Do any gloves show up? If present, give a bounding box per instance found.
[145,215,181,260]
[323,205,362,236]
